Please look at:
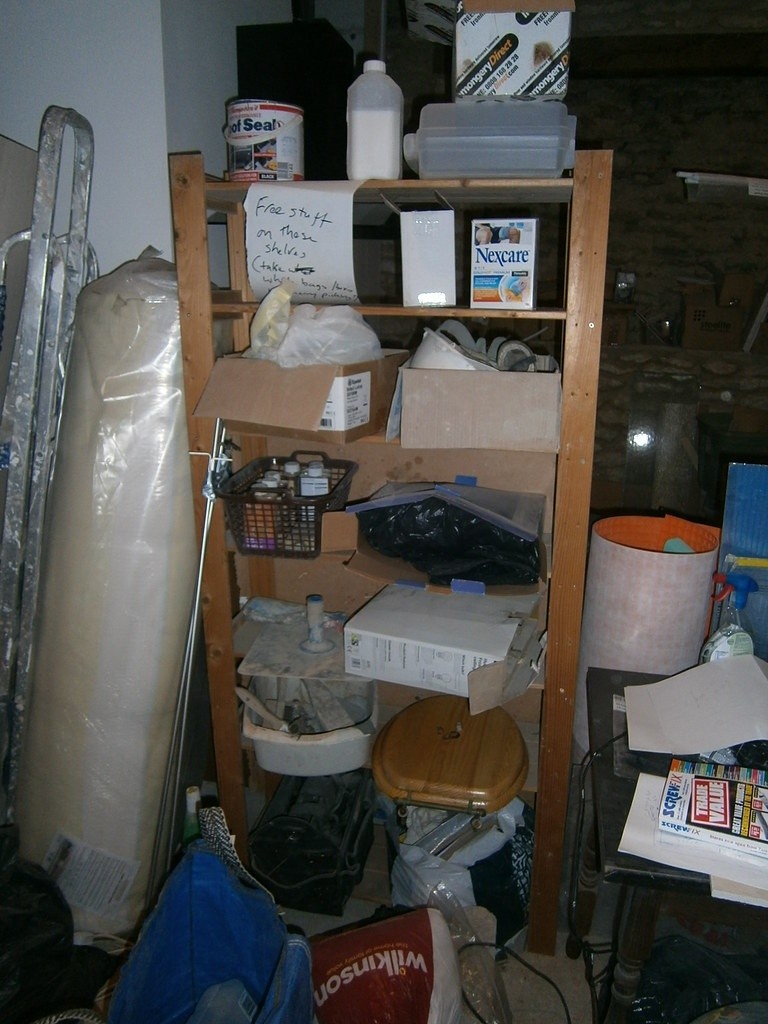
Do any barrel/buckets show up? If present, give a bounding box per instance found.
[226,96,303,181]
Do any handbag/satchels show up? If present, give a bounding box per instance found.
[108,808,313,1024]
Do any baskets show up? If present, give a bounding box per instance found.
[215,450,359,558]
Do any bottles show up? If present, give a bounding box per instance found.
[347,61,400,182]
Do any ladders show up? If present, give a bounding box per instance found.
[0,104,101,847]
